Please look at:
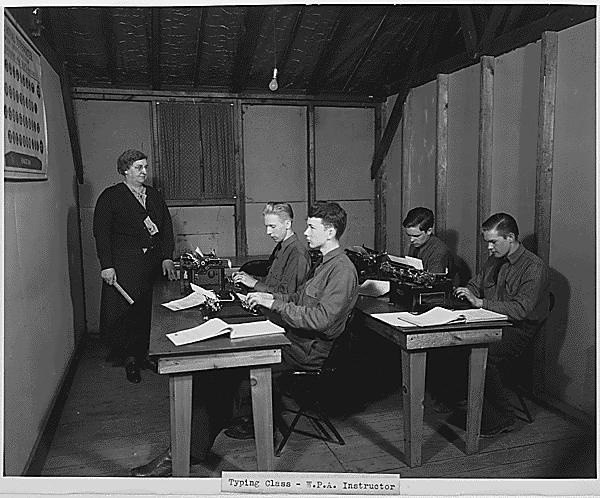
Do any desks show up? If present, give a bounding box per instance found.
[350,281,512,469]
[146,267,292,479]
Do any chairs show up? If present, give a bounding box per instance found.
[236,261,355,452]
[449,268,460,288]
[451,292,556,423]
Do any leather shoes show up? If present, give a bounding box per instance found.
[478,415,515,438]
[130,446,172,477]
[224,424,254,440]
[141,359,157,373]
[125,361,141,383]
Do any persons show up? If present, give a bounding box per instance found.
[130,200,359,476]
[93,150,178,383]
[224,202,312,439]
[434,214,550,435]
[402,207,452,273]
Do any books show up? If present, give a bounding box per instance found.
[359,279,390,297]
[166,317,286,346]
[161,292,205,311]
[398,306,508,328]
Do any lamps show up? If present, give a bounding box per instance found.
[267,6,279,92]
